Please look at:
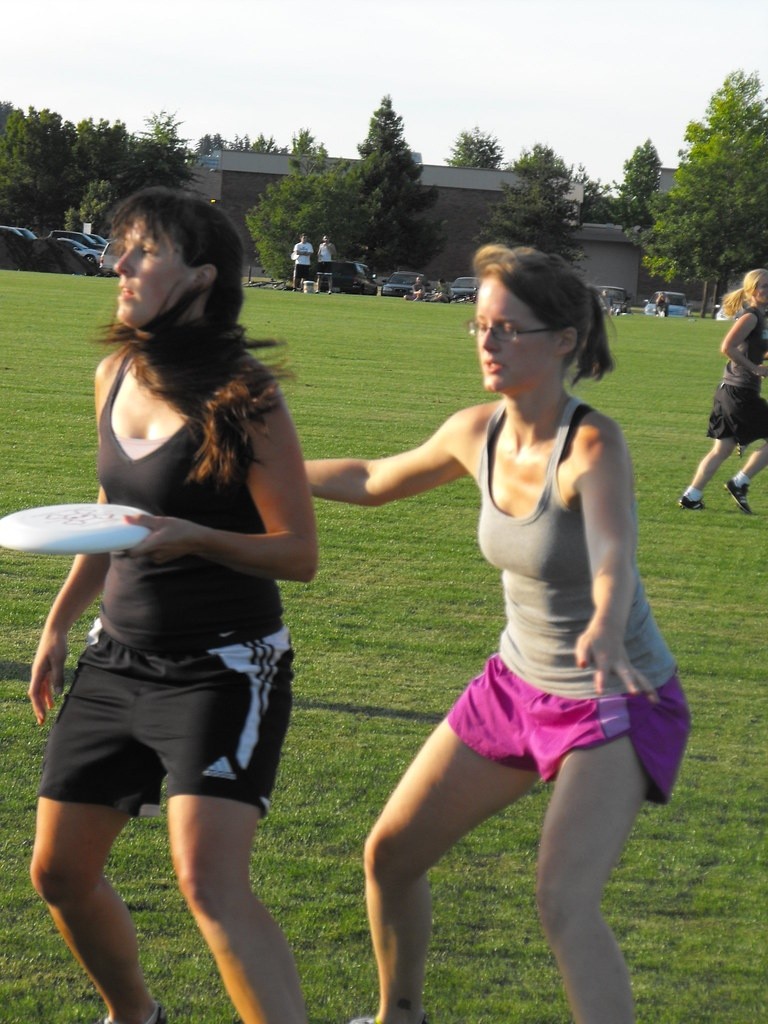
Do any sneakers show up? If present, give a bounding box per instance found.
[723,479,752,514]
[679,492,705,510]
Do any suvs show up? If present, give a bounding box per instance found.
[331,261,379,295]
[47,230,106,253]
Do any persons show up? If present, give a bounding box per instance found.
[292,233,314,291]
[315,235,335,294]
[680,269,768,514]
[655,293,668,318]
[26,190,320,1024]
[404,277,454,303]
[304,246,688,1024]
[609,303,633,316]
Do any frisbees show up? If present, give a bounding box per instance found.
[0,504,154,555]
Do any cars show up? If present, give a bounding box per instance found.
[87,234,108,246]
[100,241,125,276]
[0,226,38,241]
[381,271,427,297]
[56,238,104,266]
[644,292,693,317]
[596,286,633,314]
[449,277,480,302]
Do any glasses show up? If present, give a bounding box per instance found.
[468,322,560,341]
